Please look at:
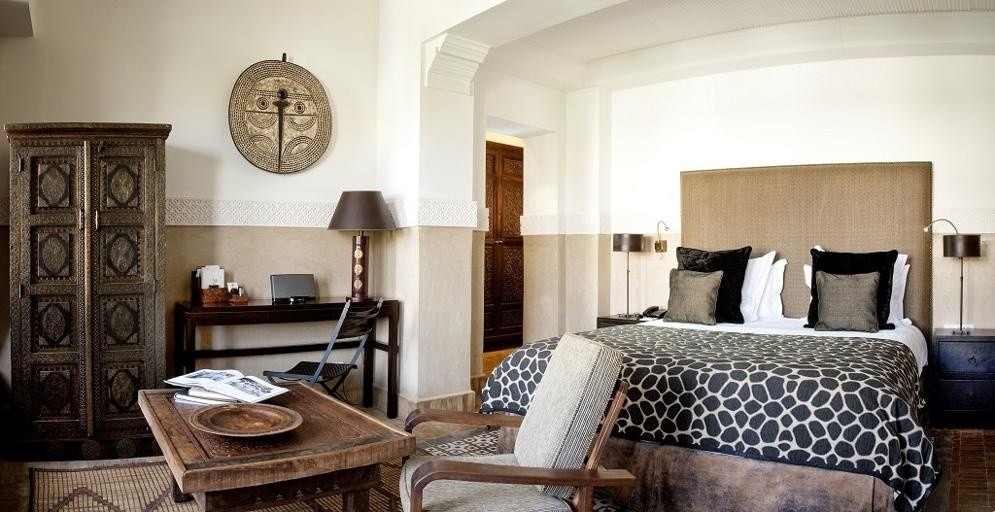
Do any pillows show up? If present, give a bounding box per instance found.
[663,246,911,332]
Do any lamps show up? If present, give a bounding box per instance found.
[329,191,396,298]
[613,234,642,316]
[943,234,980,330]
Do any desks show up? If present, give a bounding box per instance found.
[174,300,400,418]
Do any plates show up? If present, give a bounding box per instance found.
[187,403,303,440]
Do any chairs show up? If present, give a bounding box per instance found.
[399,331,637,511]
[263,297,384,405]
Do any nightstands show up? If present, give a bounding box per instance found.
[932,328,995,429]
[597,317,637,329]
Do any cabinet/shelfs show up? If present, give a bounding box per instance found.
[3,122,172,460]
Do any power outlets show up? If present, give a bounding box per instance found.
[655,241,667,252]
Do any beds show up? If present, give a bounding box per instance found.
[480,161,932,511]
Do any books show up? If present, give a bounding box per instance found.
[161,366,291,408]
